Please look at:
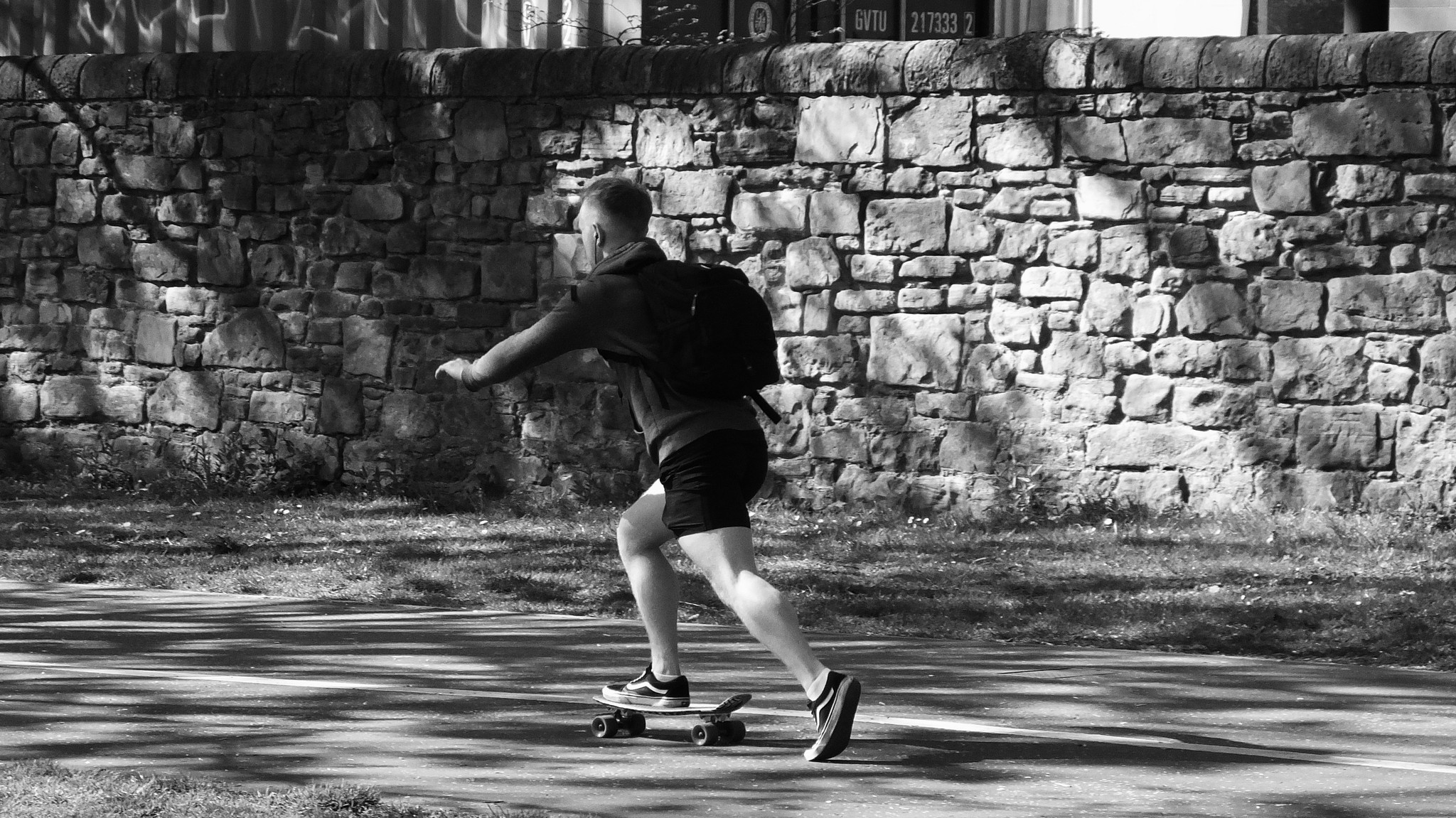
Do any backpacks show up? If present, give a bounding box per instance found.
[598,260,783,422]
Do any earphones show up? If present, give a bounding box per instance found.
[595,233,599,239]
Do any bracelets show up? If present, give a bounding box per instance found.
[461,358,487,392]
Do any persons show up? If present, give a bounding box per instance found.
[435,177,861,761]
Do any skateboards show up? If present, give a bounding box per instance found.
[591,694,752,745]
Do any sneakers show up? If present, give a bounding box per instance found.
[803,670,862,763]
[601,663,691,710]
